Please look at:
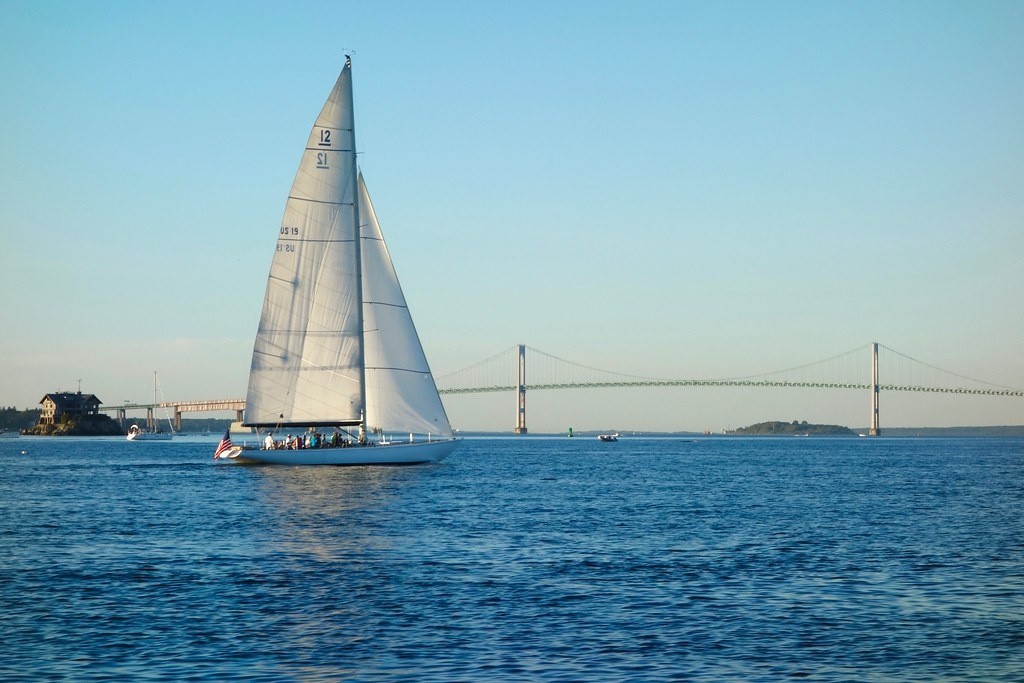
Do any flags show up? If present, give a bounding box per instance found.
[214,428,233,461]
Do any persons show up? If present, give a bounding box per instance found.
[359,430,368,446]
[264,431,347,449]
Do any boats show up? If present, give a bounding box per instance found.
[598,432,620,442]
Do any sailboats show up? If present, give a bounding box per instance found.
[214,53,463,466]
[127,371,176,440]
